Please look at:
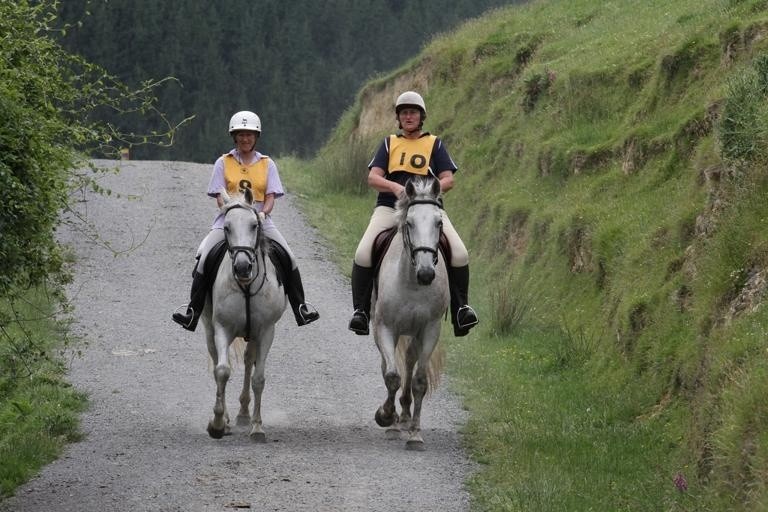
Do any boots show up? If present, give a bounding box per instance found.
[446,264,479,337]
[350,260,375,336]
[172,271,210,332]
[283,267,320,327]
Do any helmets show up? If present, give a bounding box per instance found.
[396,91,426,113]
[229,110,261,137]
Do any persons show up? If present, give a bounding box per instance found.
[348,90,478,337]
[119,144,130,160]
[172,110,320,332]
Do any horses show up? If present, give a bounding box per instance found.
[194,183,292,444]
[366,175,455,452]
